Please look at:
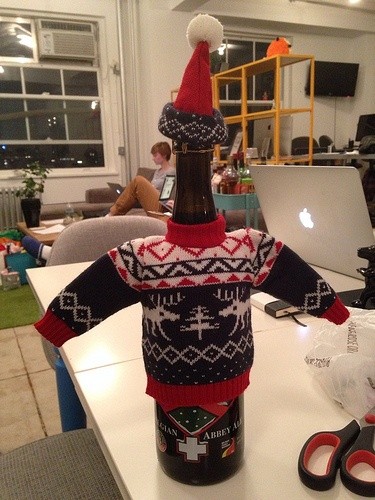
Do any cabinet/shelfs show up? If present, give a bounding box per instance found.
[171,53,315,166]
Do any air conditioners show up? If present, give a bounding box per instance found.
[35,19,97,62]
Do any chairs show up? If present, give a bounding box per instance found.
[319,135,336,166]
[291,136,322,166]
[0,427,124,500]
[38,215,167,373]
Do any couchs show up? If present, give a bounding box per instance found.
[41,168,160,219]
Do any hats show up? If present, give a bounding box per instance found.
[157,14,229,145]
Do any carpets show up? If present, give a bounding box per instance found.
[5,252,41,284]
[0,283,43,330]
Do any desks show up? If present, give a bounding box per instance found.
[25,253,374,499]
[214,192,261,230]
[302,152,375,203]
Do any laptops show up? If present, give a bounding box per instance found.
[249,165,375,281]
[107,183,123,196]
[159,175,175,213]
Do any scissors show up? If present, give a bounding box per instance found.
[298,404,375,497]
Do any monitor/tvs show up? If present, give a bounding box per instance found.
[254,116,294,159]
[356,114,375,141]
[306,61,359,97]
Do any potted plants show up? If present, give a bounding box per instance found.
[15,160,50,228]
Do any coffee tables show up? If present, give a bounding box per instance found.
[16,218,66,261]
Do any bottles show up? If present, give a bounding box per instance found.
[66,201,73,220]
[156,146,244,485]
[211,152,255,195]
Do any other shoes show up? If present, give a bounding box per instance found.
[22,236,47,263]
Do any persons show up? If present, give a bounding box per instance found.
[105,144,176,223]
[82,142,171,218]
